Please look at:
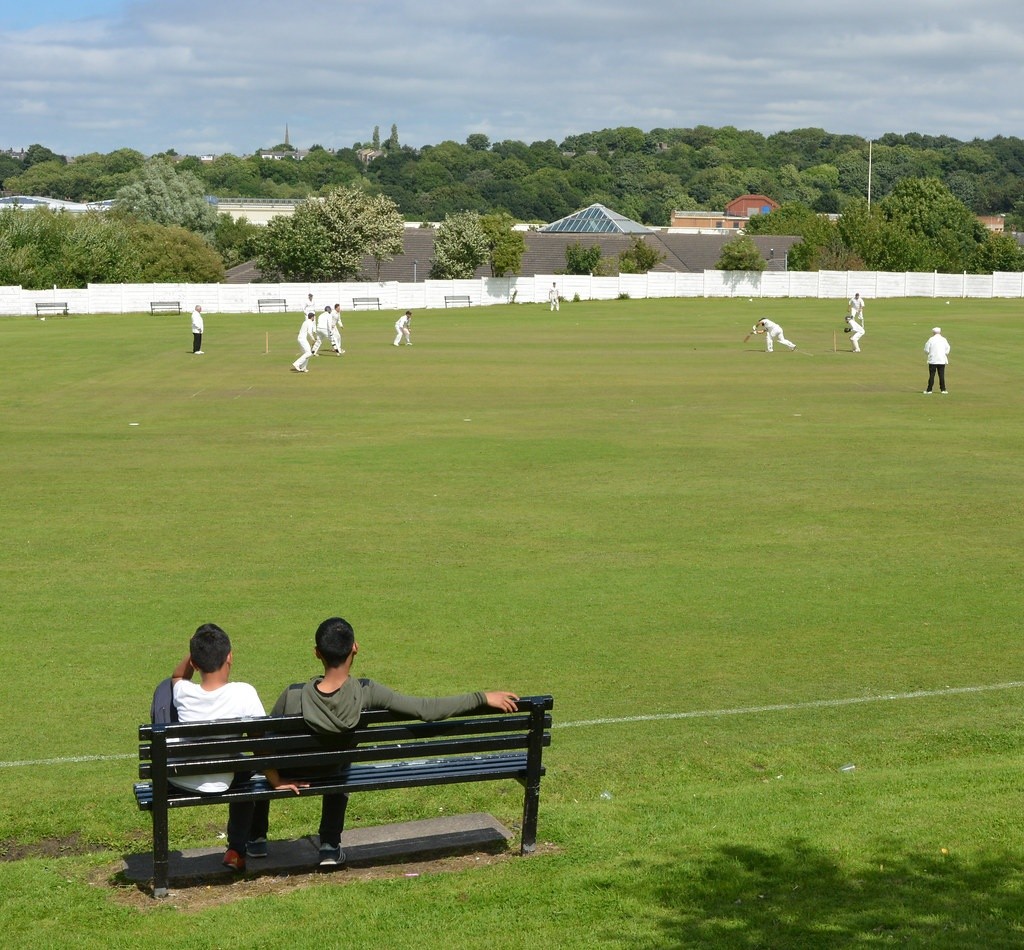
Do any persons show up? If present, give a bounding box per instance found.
[844,316,866,352]
[311,306,341,358]
[847,292,865,329]
[246,617,520,871]
[548,282,560,312]
[149,624,311,871]
[922,326,951,395]
[393,310,413,347]
[190,305,205,354]
[328,304,346,354]
[753,317,797,353]
[303,292,317,334]
[293,312,314,373]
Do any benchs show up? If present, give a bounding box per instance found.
[132,695,554,900]
[35,302,69,317]
[258,298,288,313]
[353,297,383,311]
[150,301,183,316]
[444,295,473,309]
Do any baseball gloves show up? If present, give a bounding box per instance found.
[844,328,850,332]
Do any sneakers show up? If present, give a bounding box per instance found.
[245,837,268,857]
[221,849,245,870]
[318,843,347,865]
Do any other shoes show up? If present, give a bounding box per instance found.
[766,350,773,352]
[199,351,204,354]
[853,349,860,352]
[394,343,399,346]
[406,343,412,346]
[941,391,948,394]
[292,364,300,371]
[792,345,796,351]
[300,369,309,372]
[924,391,933,394]
[337,350,345,356]
[194,351,200,354]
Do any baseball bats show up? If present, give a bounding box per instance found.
[744,329,754,342]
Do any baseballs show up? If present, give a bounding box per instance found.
[694,348,696,350]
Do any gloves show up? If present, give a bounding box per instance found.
[753,331,757,334]
[753,325,757,330]
